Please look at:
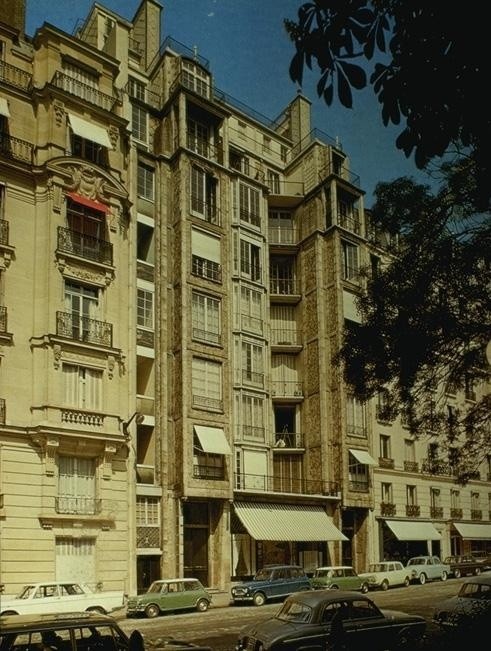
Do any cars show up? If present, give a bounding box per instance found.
[128,577,213,620]
[1,581,126,619]
[431,575,491,639]
[232,550,491,607]
[234,589,426,649]
[1,613,211,651]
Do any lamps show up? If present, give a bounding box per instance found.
[119,409,145,437]
